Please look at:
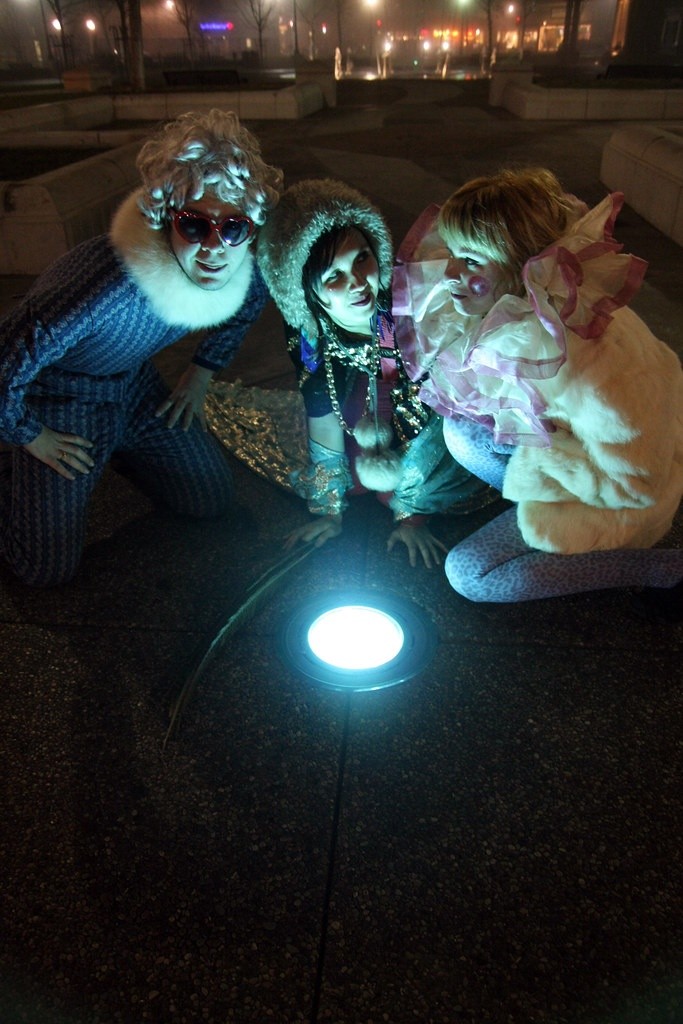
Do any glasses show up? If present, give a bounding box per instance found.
[166,207,253,248]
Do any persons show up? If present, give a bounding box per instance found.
[394,169,683,604]
[258,180,489,573]
[0,107,284,587]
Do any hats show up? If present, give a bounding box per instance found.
[256,178,394,349]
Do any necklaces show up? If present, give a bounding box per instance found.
[324,325,380,437]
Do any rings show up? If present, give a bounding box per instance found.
[60,452,66,460]
